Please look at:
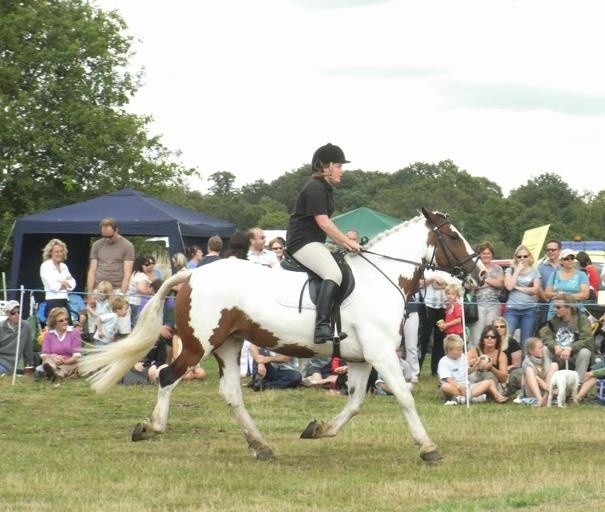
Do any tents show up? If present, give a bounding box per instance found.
[9,189,236,319]
[334,208,402,239]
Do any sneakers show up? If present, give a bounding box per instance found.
[302,374,338,389]
[33,357,68,383]
[495,393,510,405]
[472,392,488,404]
[247,374,266,392]
[373,388,389,396]
[454,394,467,406]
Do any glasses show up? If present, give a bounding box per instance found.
[101,235,115,240]
[546,248,558,252]
[551,304,563,310]
[57,316,70,323]
[143,264,149,267]
[272,246,283,251]
[516,254,529,258]
[492,324,507,329]
[8,309,21,315]
[564,257,576,261]
[483,334,498,340]
[150,261,155,265]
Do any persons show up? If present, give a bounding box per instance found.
[4,219,605,404]
[287,143,364,343]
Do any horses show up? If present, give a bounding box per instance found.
[74,206,488,462]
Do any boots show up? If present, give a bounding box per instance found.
[311,277,348,349]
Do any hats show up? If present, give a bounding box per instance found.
[309,140,353,168]
[560,247,578,259]
[4,299,21,311]
